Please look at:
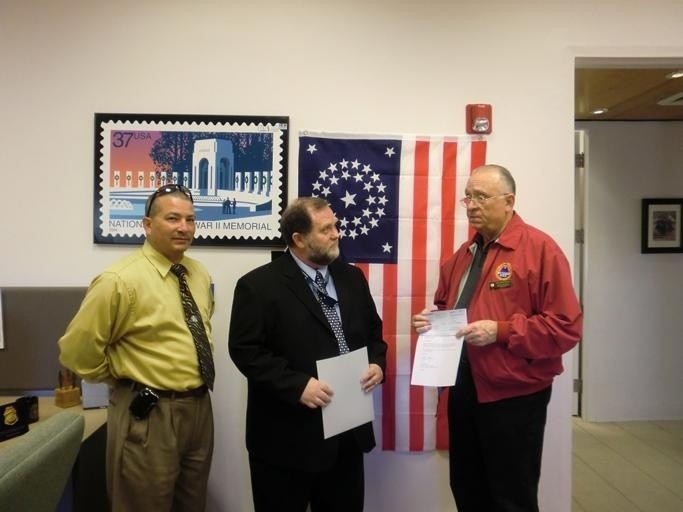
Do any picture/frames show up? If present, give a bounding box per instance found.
[93,112,290,248]
[641,199,683,253]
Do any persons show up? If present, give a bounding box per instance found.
[226,193,391,512]
[412,163,583,511]
[57,184,215,512]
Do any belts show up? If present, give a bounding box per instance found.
[113,379,210,398]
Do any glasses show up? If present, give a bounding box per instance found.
[463,193,506,204]
[145,184,193,218]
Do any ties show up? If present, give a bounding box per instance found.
[170,264,216,394]
[314,271,349,355]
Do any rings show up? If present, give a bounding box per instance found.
[375,381,378,385]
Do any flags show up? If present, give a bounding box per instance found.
[295,130,490,455]
